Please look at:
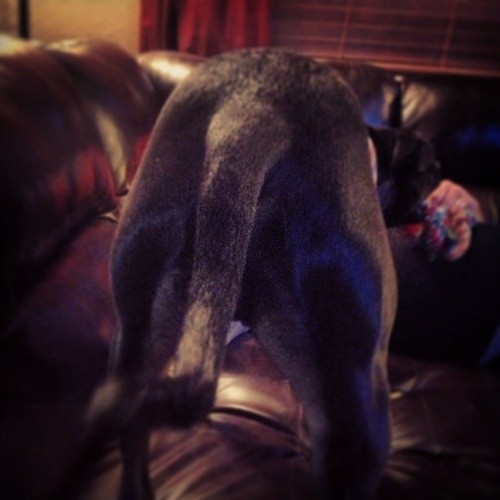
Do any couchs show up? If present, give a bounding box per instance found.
[1,34,500,500]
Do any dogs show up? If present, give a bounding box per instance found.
[55,44,400,500]
